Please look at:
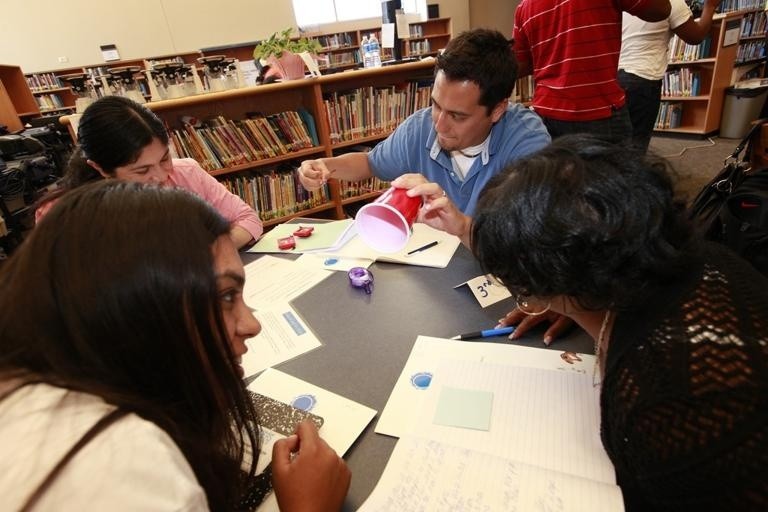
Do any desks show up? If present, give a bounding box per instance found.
[235,217,601,510]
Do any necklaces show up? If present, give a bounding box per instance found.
[590,302,615,389]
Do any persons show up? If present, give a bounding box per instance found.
[34,95,262,250]
[297,26,552,257]
[621,1,718,158]
[0,178,353,510]
[467,134,767,510]
[512,0,671,147]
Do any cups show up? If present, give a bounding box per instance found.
[353,185,426,252]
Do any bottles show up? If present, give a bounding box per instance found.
[360,32,381,70]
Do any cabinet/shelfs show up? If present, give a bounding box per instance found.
[653,0,767,141]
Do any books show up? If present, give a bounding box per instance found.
[305,27,534,222]
[330,221,461,269]
[355,343,624,512]
[654,0,768,131]
[23,55,329,220]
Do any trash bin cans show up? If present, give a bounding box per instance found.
[718,86,767,140]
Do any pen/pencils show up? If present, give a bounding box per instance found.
[450,327,515,342]
[408,241,439,254]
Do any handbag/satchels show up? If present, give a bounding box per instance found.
[689,155,749,231]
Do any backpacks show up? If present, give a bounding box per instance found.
[719,168,768,256]
